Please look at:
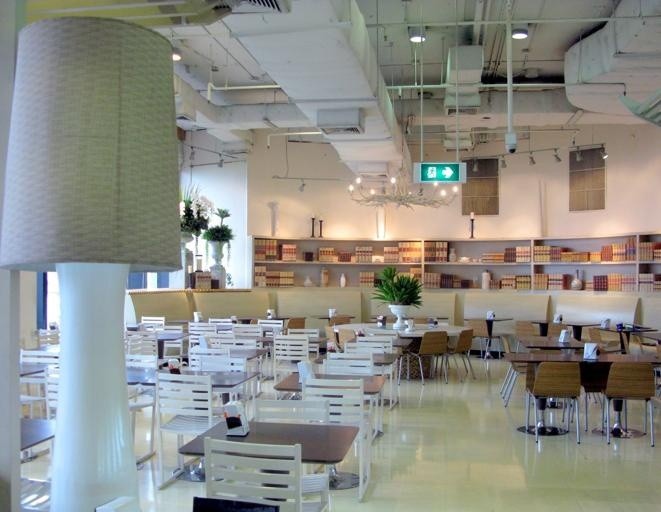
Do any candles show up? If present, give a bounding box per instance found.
[470,211,475,219]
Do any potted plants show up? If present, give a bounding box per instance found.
[178,190,235,264]
[369,268,424,330]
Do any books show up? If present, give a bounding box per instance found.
[383,242,423,264]
[640,240,661,261]
[534,240,636,262]
[424,241,448,263]
[425,273,469,289]
[534,273,571,290]
[638,273,661,293]
[584,273,637,292]
[490,275,531,290]
[360,271,374,288]
[482,247,531,263]
[317,246,373,263]
[193,271,212,290]
[395,268,422,286]
[319,266,329,287]
[255,239,296,288]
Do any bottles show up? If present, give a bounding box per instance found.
[319,265,328,287]
[339,272,345,288]
[481,268,490,289]
[472,271,480,288]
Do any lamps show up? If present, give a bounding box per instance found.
[0,18,182,512]
[408,26,427,43]
[272,175,341,191]
[465,127,609,172]
[182,142,238,168]
[510,22,530,40]
[346,66,459,212]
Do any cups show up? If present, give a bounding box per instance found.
[304,251,313,261]
[329,308,336,319]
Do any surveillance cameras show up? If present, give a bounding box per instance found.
[504,133,517,154]
